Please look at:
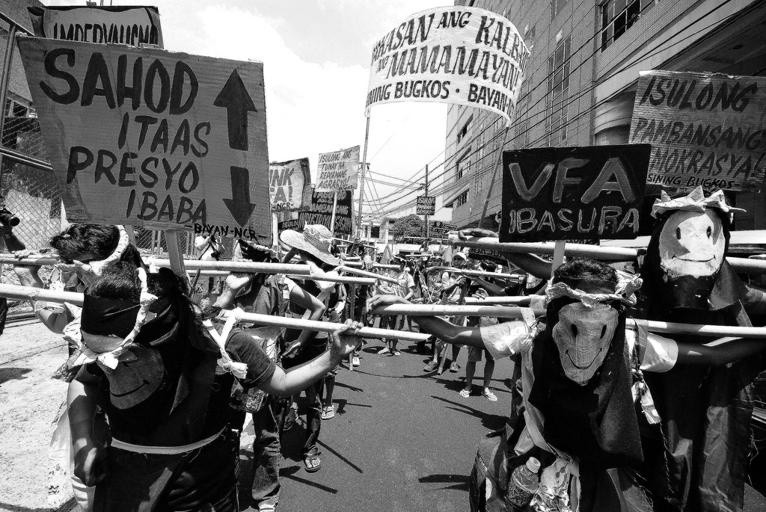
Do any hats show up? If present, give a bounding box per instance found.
[279,224,339,266]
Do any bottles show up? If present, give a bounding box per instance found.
[503,455,541,512]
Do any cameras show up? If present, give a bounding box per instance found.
[0,206,21,230]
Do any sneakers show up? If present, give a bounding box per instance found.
[352,356,361,367]
[450,362,458,373]
[423,360,438,372]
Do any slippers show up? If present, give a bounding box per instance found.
[459,389,473,399]
[321,405,334,420]
[302,455,321,473]
[480,390,497,402]
